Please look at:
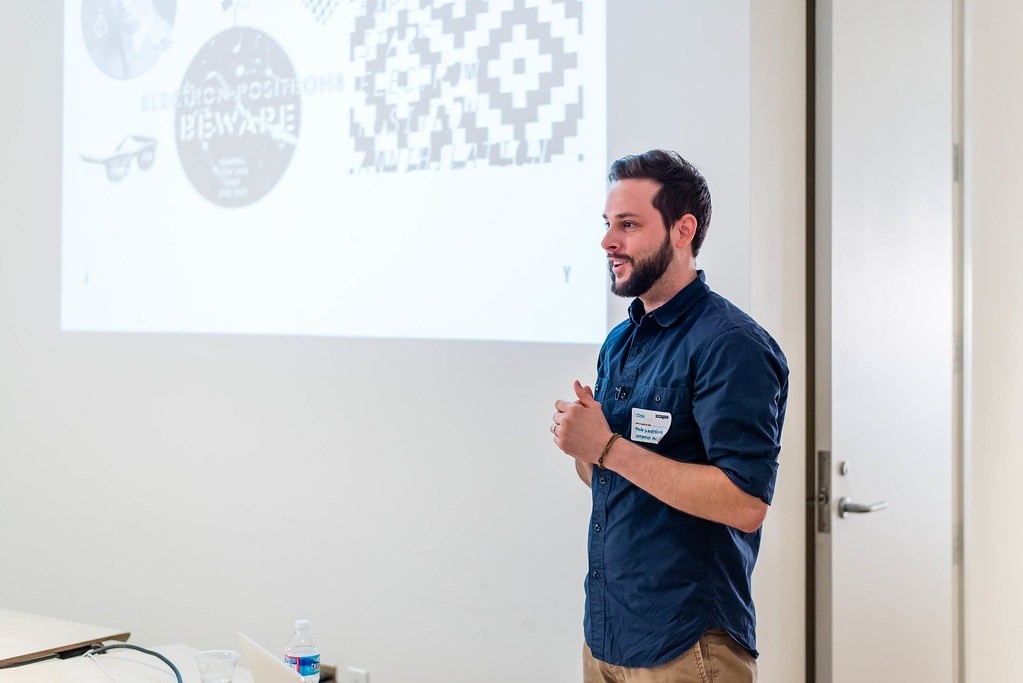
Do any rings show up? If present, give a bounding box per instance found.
[552,424,558,433]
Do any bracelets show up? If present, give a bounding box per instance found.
[598,434,622,469]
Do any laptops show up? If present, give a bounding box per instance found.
[0,607,131,669]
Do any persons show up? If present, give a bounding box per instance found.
[550,149,791,683]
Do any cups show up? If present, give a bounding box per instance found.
[194,650,241,683]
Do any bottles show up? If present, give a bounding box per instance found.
[283,619,320,683]
